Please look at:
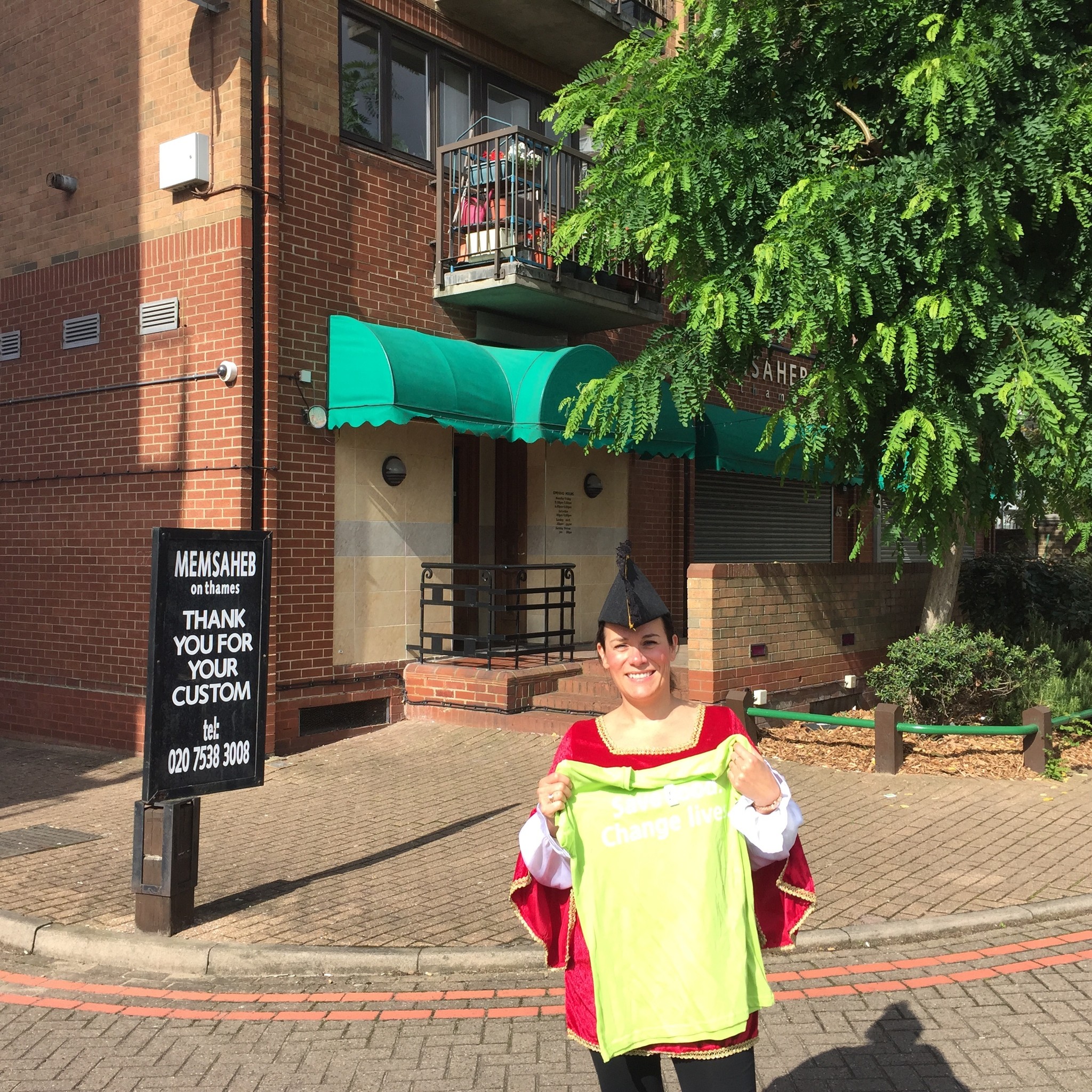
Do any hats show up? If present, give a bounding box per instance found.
[597,540,669,633]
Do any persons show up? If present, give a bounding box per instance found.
[509,538,819,1092]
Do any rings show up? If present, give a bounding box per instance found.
[548,792,555,803]
[733,755,741,764]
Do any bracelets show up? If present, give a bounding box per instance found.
[752,790,783,812]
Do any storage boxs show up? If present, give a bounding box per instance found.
[465,158,519,185]
[463,227,518,262]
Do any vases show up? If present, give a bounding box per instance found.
[531,248,554,270]
[454,244,472,265]
[489,198,513,222]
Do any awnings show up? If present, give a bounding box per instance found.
[325,310,1009,503]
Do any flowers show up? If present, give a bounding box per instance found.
[526,214,558,253]
[481,140,542,171]
